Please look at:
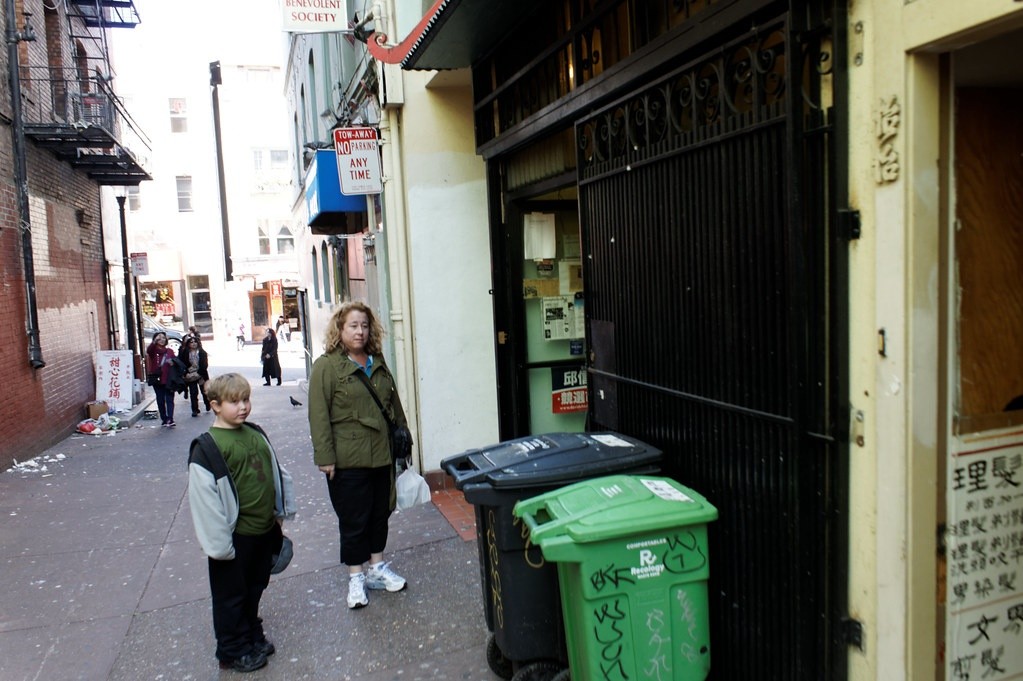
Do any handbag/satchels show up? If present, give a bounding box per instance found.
[186,367,200,382]
[395,457,431,511]
[389,423,413,459]
[147,367,162,386]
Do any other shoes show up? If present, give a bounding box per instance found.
[192,409,200,417]
[161,420,176,427]
[250,637,275,656]
[206,404,211,411]
[219,647,266,672]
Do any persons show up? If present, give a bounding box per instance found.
[261,328,281,386]
[147,332,176,428]
[187,372,295,671]
[178,327,211,416]
[276,316,290,342]
[235,318,245,351]
[309,301,412,609]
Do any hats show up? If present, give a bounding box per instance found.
[271,535,294,574]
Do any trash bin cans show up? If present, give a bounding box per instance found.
[514,475,718,681]
[438,432,662,681]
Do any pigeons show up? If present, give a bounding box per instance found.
[290,396,302,407]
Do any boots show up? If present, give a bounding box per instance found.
[264,377,270,386]
[276,377,282,386]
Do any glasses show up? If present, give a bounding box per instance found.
[190,341,198,344]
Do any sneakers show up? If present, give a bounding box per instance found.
[347,572,368,608]
[366,561,408,592]
